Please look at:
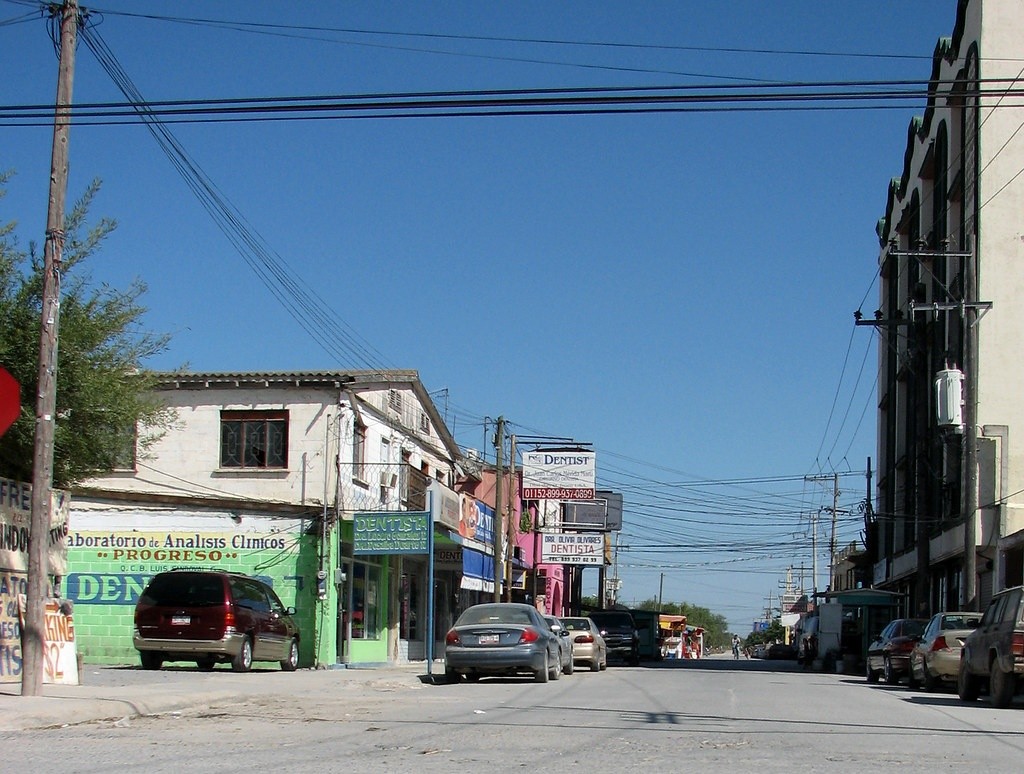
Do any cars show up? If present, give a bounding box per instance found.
[906,610,985,692]
[865,618,958,685]
[442,600,563,684]
[745,643,798,661]
[557,616,609,672]
[513,614,575,675]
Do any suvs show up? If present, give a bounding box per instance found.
[131,566,301,673]
[583,609,643,667]
[794,616,862,667]
[957,583,1024,710]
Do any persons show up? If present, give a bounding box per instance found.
[731,635,740,659]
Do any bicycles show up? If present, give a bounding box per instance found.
[732,644,740,659]
